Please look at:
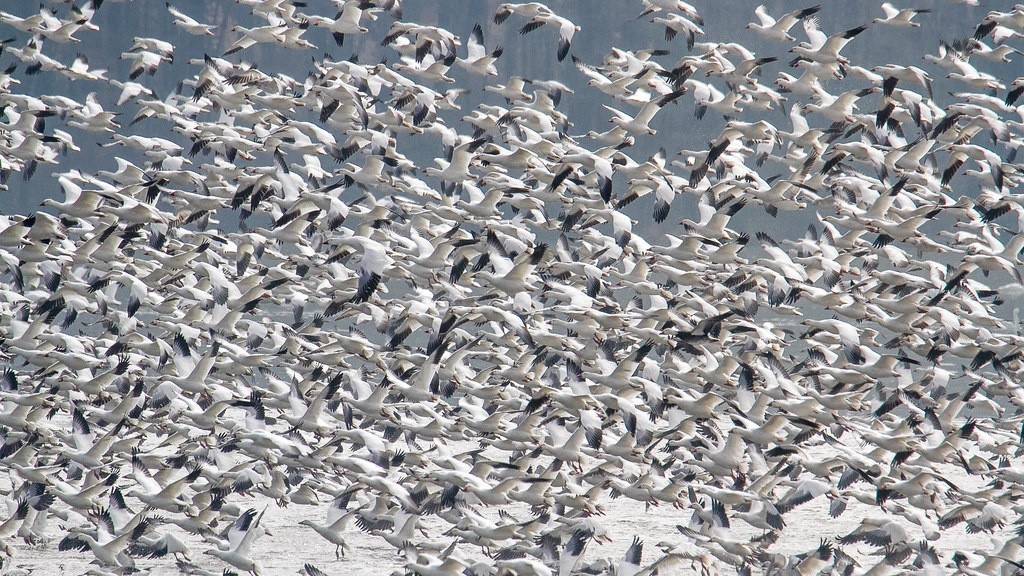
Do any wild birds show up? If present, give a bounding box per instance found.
[0,0,1024,576]
